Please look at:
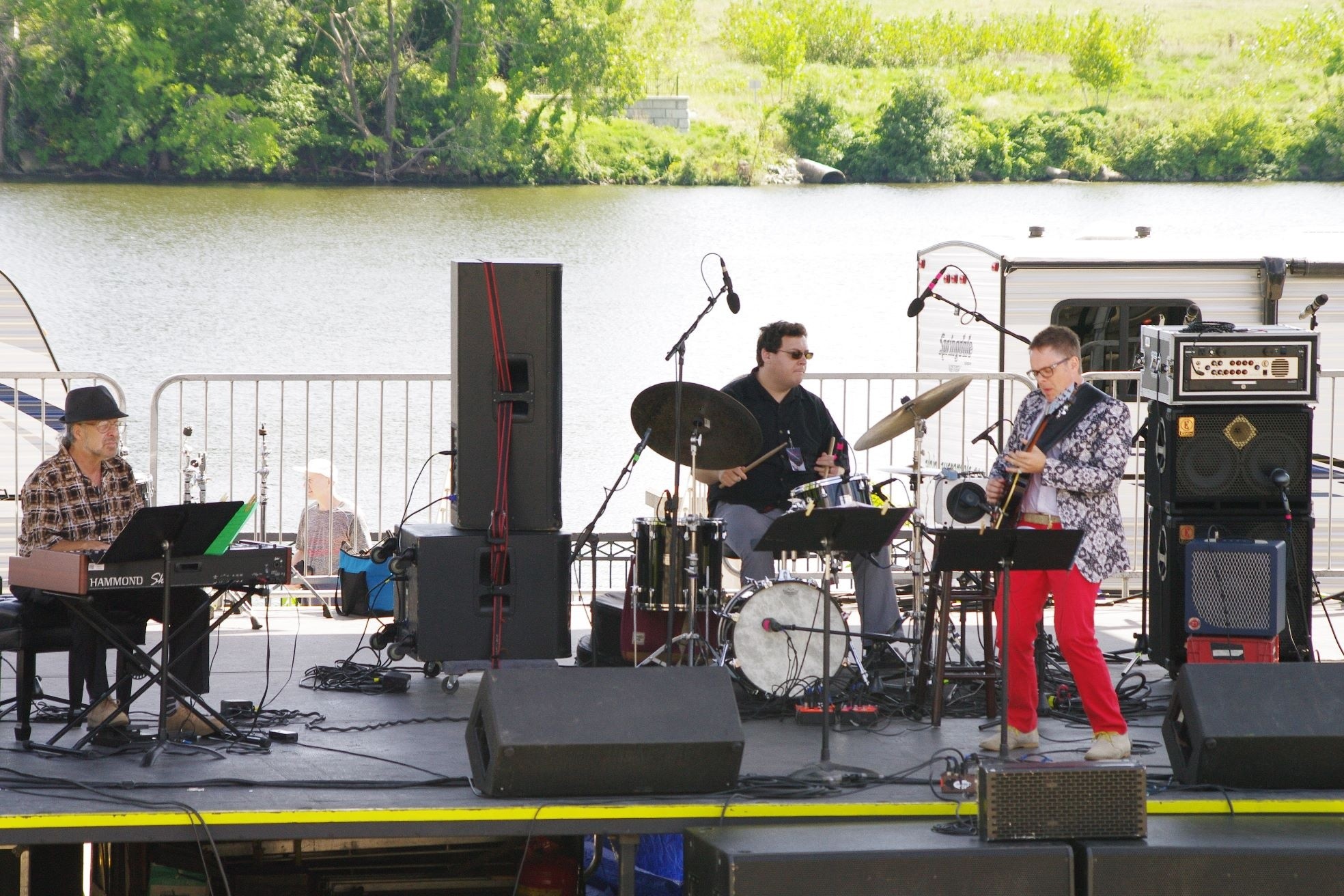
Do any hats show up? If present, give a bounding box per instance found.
[58,385,129,423]
[296,459,337,481]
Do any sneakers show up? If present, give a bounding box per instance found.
[1084,731,1130,760]
[166,704,223,738]
[978,724,1039,751]
[86,694,129,730]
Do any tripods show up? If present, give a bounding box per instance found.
[1100,423,1153,678]
[96,502,243,766]
[634,437,738,681]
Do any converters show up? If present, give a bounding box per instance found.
[269,730,298,742]
[382,672,411,692]
[219,699,253,717]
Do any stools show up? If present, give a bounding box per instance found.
[0,595,147,743]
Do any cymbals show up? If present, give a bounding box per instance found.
[629,380,764,471]
[875,468,941,476]
[852,371,975,452]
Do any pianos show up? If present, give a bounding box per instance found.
[7,539,293,760]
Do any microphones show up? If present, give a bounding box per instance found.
[1183,304,1199,325]
[1300,294,1328,319]
[1269,469,1293,520]
[720,257,740,314]
[971,421,1005,444]
[907,268,947,316]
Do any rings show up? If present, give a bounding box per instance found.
[829,467,832,474]
[1018,468,1022,474]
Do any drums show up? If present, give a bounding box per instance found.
[717,573,853,702]
[790,473,876,555]
[630,512,727,611]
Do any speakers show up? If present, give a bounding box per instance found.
[449,262,562,532]
[1145,400,1313,509]
[1184,536,1289,636]
[390,523,571,663]
[1144,500,1316,662]
[466,667,744,796]
[1161,661,1344,791]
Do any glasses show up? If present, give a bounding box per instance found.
[76,420,128,434]
[1026,359,1069,380]
[775,349,814,360]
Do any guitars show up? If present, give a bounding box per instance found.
[992,397,1074,530]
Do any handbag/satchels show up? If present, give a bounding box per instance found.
[335,540,394,616]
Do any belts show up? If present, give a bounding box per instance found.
[1020,513,1060,526]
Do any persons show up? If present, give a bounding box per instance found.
[290,458,369,607]
[10,387,227,742]
[977,326,1131,762]
[690,322,907,670]
